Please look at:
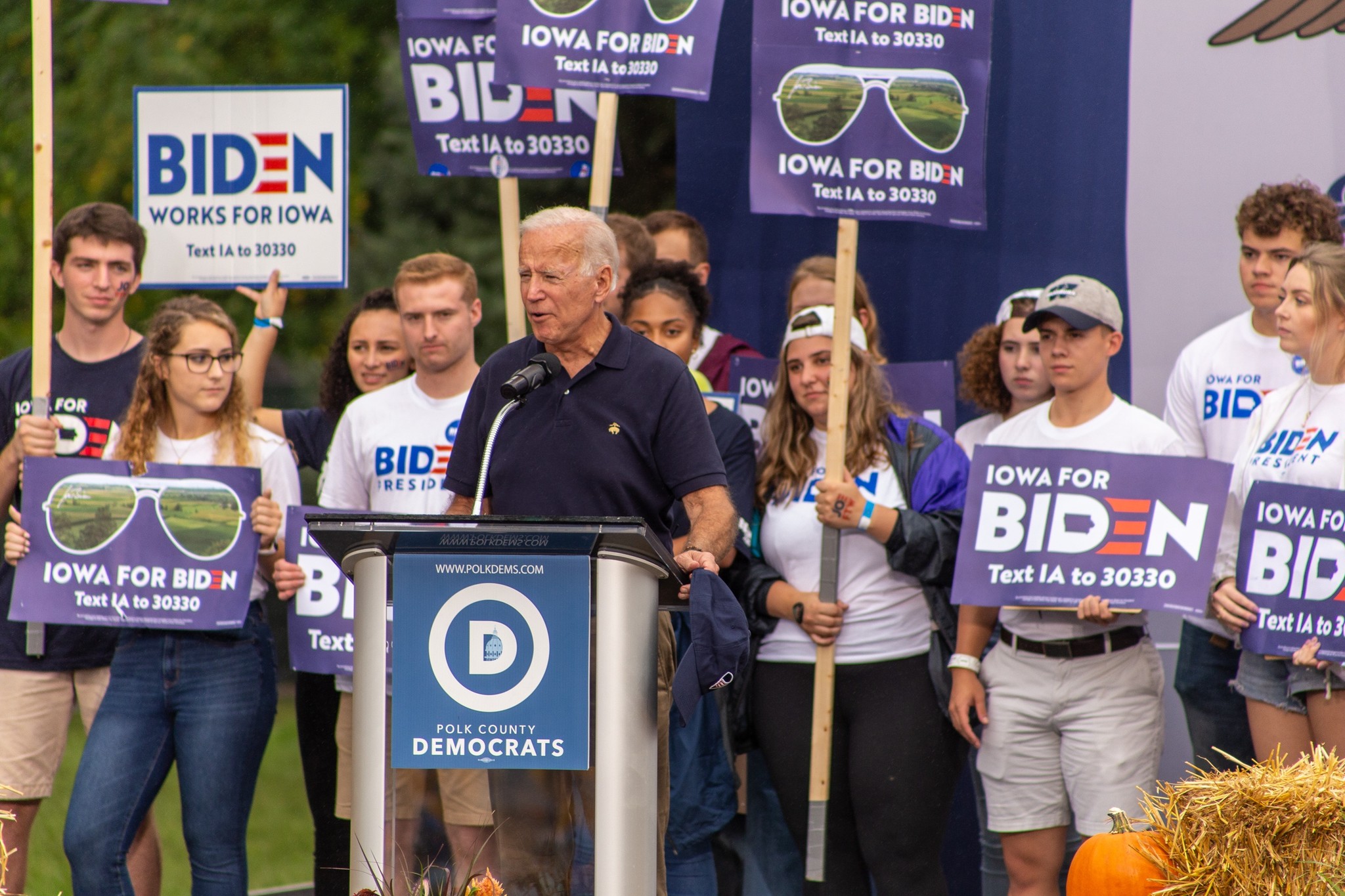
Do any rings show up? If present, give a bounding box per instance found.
[1216,613,1221,619]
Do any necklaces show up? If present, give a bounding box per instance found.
[1053,400,1091,428]
[1302,375,1334,428]
[58,327,131,355]
[167,423,197,465]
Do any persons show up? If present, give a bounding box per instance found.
[443,206,737,896]
[743,307,982,896]
[1163,179,1345,777]
[1208,241,1345,767]
[4,296,299,896]
[233,269,416,896]
[604,210,886,896]
[948,276,1184,896]
[0,203,162,896]
[274,254,496,896]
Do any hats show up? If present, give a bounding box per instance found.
[781,304,868,353]
[988,287,1045,325]
[673,568,751,728]
[1020,274,1124,334]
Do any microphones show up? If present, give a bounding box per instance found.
[499,351,561,399]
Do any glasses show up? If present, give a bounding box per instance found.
[160,350,244,374]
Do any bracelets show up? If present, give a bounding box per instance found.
[947,653,981,674]
[857,500,875,531]
[254,317,283,330]
[679,545,702,553]
[259,541,278,555]
[252,315,284,330]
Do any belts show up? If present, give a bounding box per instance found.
[997,622,1146,658]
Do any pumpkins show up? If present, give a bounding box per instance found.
[1066,808,1182,896]
